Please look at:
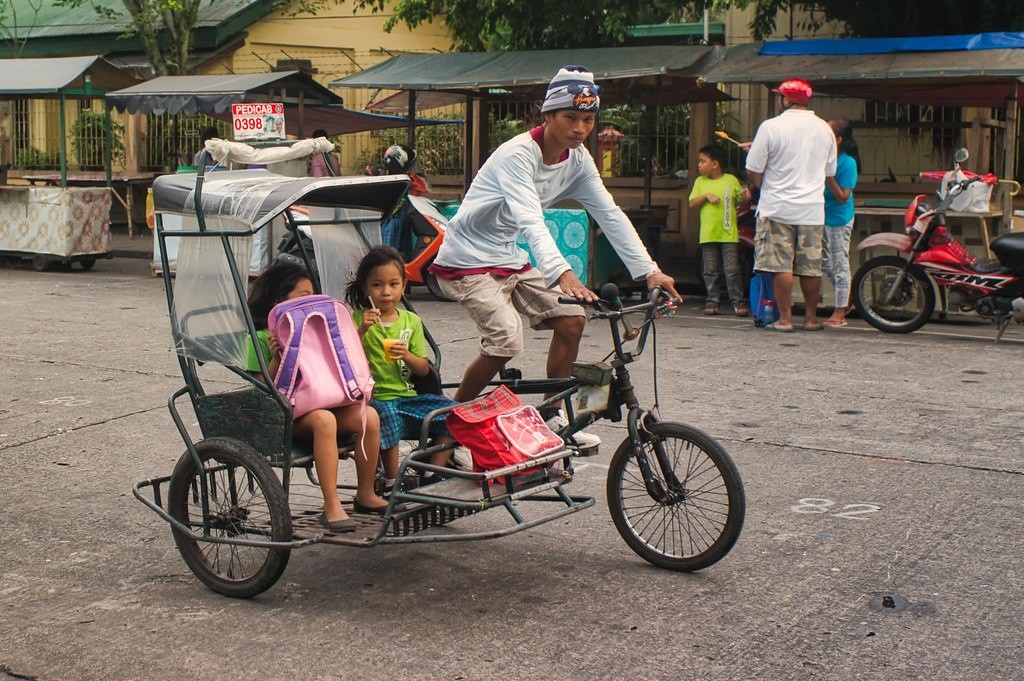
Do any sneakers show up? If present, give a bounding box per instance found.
[449,442,475,475]
[705,302,718,314]
[734,306,749,317]
[545,408,601,448]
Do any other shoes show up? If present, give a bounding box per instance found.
[353,496,406,514]
[320,504,357,532]
[419,470,444,486]
[381,481,408,504]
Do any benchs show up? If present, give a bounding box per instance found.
[183,305,436,465]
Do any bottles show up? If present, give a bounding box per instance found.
[675,170,688,178]
[764,300,773,321]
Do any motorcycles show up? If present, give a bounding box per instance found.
[850,148,1024,344]
[276,136,469,301]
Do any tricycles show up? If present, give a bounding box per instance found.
[131,136,746,601]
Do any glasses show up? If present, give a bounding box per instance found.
[544,83,599,100]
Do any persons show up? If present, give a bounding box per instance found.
[821,119,861,328]
[426,64,684,466]
[242,260,407,533]
[365,145,431,264]
[737,78,838,332]
[688,144,752,317]
[311,129,341,177]
[194,126,220,166]
[341,245,464,502]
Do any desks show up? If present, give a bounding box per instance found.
[440,206,662,297]
[855,208,1005,306]
[6,171,155,240]
[2,185,113,262]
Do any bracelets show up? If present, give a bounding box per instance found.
[646,269,661,279]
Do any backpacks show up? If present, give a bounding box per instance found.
[268,294,374,421]
[750,273,779,329]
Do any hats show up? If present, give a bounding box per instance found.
[541,65,600,119]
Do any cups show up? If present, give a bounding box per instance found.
[384,339,403,361]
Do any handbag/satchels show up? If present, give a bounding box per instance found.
[445,384,566,484]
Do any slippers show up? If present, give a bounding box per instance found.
[804,321,823,330]
[824,318,848,328]
[767,320,794,333]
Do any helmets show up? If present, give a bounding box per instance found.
[771,78,813,107]
[384,144,416,173]
